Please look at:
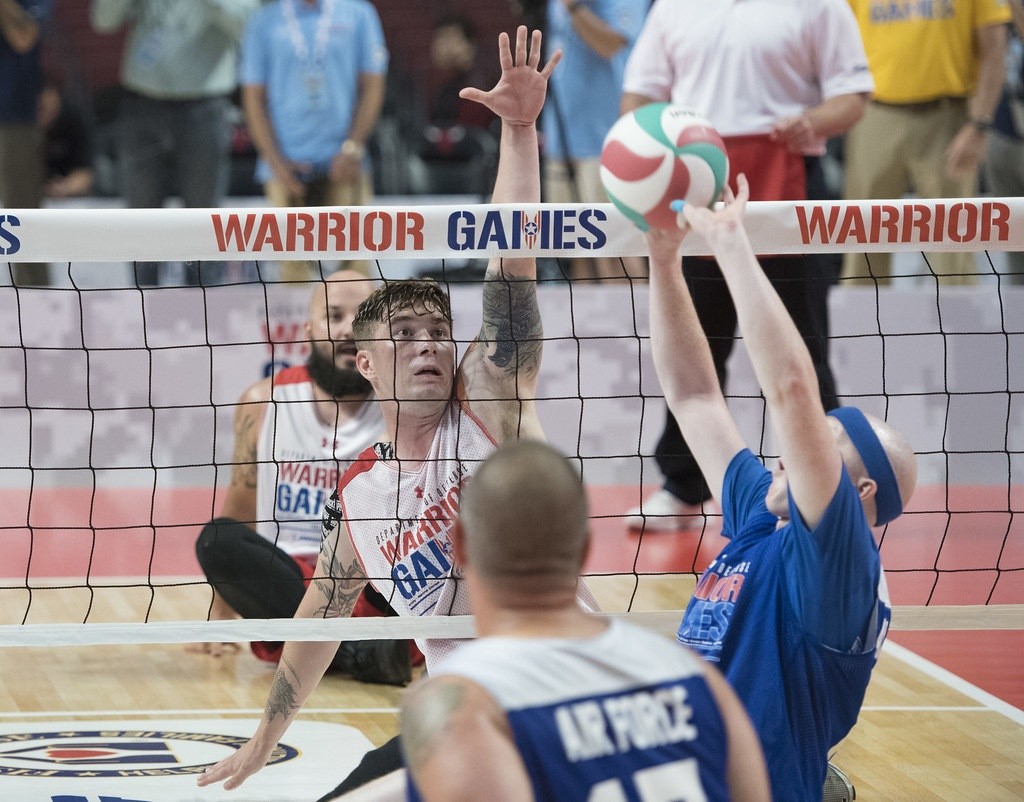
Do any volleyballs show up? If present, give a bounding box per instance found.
[599,102,731,233]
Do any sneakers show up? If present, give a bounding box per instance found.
[626,491,716,530]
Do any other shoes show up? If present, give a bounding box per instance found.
[350,639,412,686]
[823,750,856,802]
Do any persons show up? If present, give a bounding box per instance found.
[0,0,1024,290]
[649,173,916,802]
[401,441,771,802]
[621,0,872,533]
[195,25,605,789]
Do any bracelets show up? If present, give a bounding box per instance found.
[963,114,992,133]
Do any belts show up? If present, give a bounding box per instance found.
[870,97,968,109]
